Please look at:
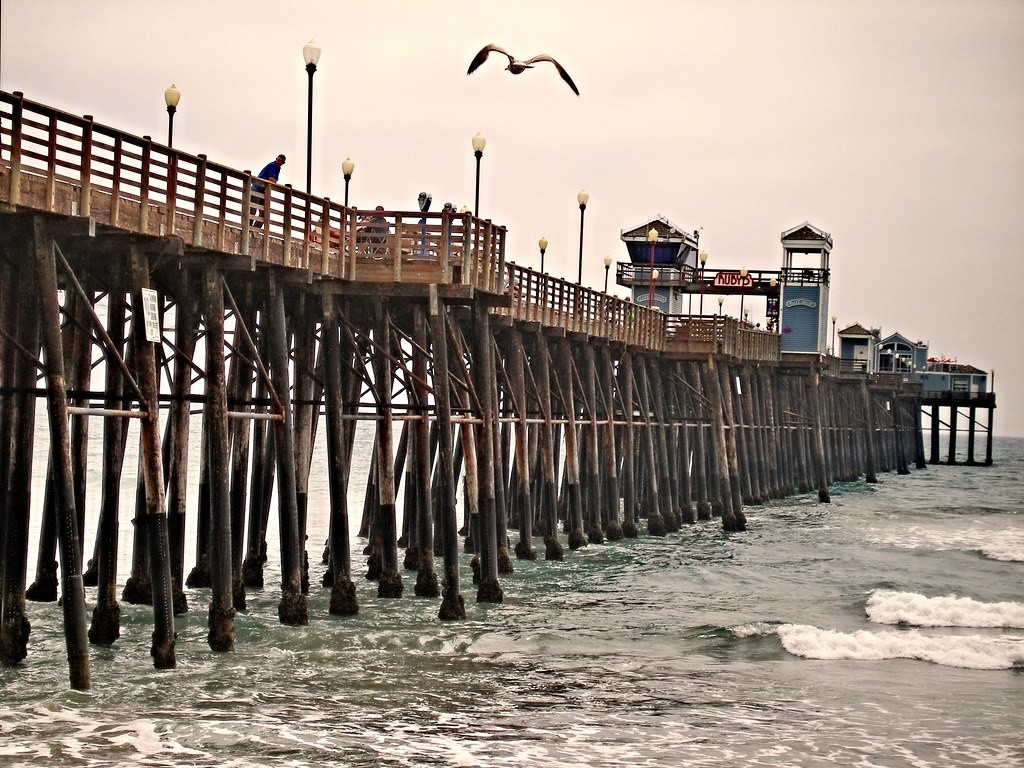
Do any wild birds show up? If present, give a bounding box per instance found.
[466,43,580,96]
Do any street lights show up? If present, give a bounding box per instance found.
[472,129,486,218]
[744,305,749,322]
[603,255,612,293]
[769,276,777,333]
[831,313,837,356]
[718,296,724,315]
[341,156,354,209]
[577,189,589,285]
[651,269,659,306]
[164,82,183,148]
[699,250,708,320]
[539,237,548,274]
[740,266,748,321]
[302,39,322,194]
[648,227,658,308]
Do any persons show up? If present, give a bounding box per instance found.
[369,206,390,254]
[248,154,286,228]
